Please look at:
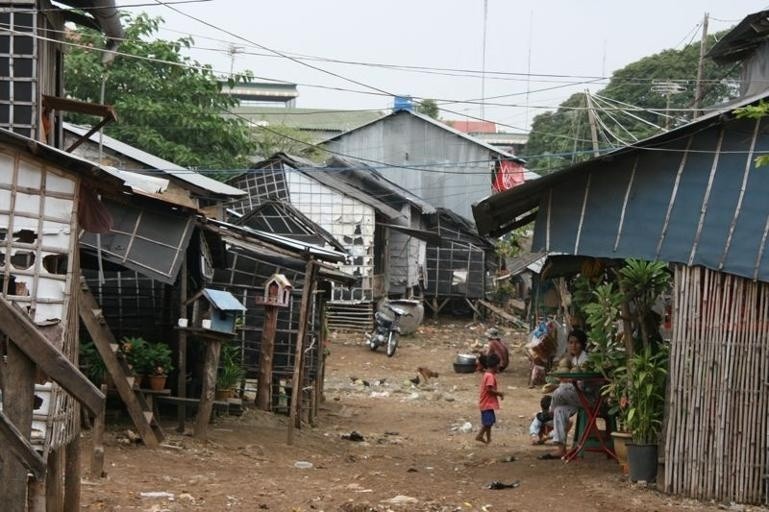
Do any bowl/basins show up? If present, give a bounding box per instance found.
[453,354,477,374]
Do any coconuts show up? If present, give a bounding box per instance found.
[557,358,582,373]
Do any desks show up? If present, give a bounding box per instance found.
[559,373,619,464]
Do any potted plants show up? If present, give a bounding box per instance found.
[216,348,247,401]
[79,336,175,391]
[574,256,673,483]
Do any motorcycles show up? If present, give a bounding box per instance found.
[366,303,413,356]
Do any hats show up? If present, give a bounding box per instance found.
[485,328,500,341]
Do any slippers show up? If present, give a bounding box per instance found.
[536,412,554,422]
[341,430,365,442]
[537,452,560,460]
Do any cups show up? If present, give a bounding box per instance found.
[202,319,211,329]
[178,318,188,328]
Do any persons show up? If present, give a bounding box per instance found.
[474,354,504,444]
[477,326,510,372]
[528,327,588,460]
